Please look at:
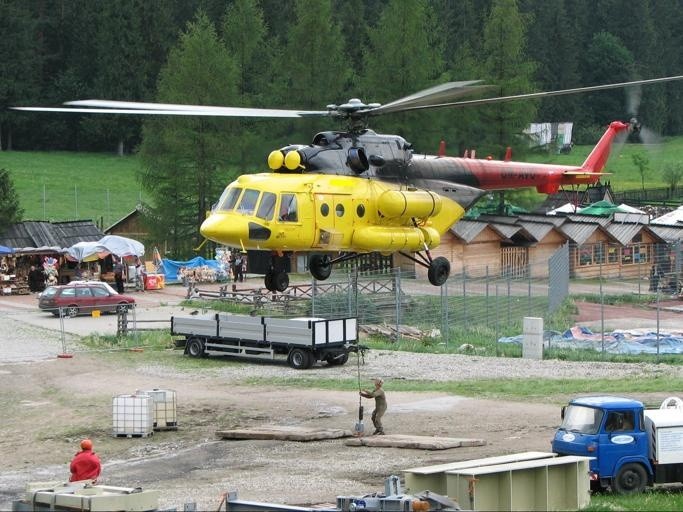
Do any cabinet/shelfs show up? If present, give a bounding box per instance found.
[399,450,559,498]
[446,455,597,512]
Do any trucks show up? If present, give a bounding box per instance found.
[551,396,681,496]
[515,120,575,154]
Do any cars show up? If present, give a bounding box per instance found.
[37,283,136,317]
[36,279,120,299]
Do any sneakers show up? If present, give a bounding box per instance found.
[372,428,385,435]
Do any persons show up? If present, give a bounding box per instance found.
[359,378,387,434]
[648,261,665,292]
[114,259,123,293]
[69,440,101,482]
[28,266,42,291]
[135,259,145,292]
[229,253,243,282]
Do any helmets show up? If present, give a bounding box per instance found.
[80,439,93,450]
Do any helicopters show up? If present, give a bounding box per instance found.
[7,73,682,293]
